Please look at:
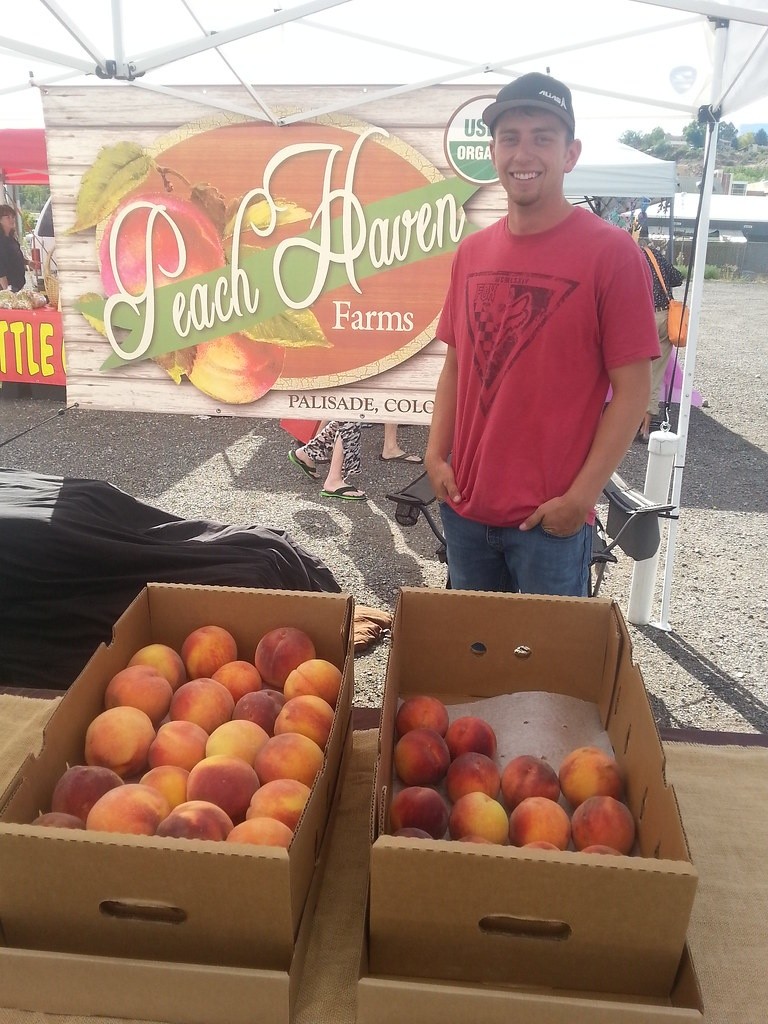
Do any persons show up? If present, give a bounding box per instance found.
[0,204,41,293]
[379,424,424,464]
[628,227,683,442]
[289,421,366,500]
[426,72,663,597]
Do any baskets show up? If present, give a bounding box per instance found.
[44,247,58,308]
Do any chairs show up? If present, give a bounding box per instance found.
[385,458,678,601]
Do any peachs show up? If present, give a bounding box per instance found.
[387,695,637,858]
[98,194,285,403]
[27,625,343,850]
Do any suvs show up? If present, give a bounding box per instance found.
[30,195,56,290]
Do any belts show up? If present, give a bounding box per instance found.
[654,306,668,312]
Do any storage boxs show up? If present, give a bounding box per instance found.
[0,580,706,1024]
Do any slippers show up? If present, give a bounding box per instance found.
[379,451,423,465]
[638,432,648,442]
[289,450,320,481]
[321,484,366,500]
[297,439,331,463]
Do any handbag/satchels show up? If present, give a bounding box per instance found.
[668,299,690,347]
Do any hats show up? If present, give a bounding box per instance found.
[482,72,575,130]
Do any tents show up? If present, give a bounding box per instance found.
[0,0,768,630]
